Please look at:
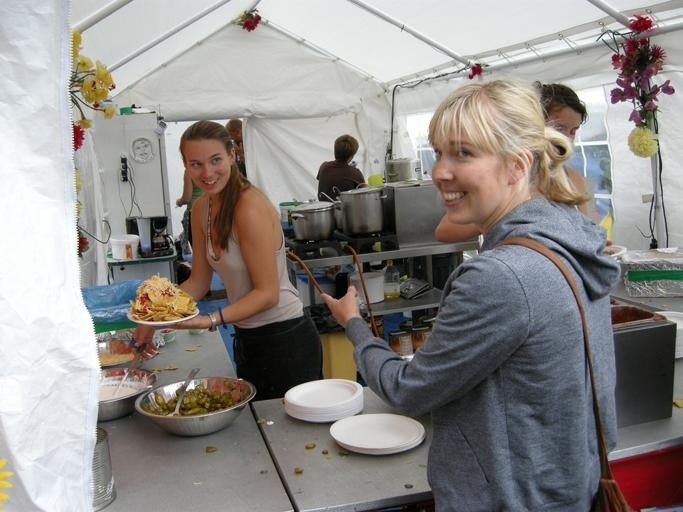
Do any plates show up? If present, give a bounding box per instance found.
[330,414,426,455]
[128,308,200,326]
[284,377,364,423]
[93,426,117,507]
[654,312,683,360]
[604,245,626,258]
[351,273,387,302]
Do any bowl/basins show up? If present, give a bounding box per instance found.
[97,367,154,421]
[98,341,138,368]
[136,377,256,438]
[109,235,140,259]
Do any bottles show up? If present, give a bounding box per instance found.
[383,259,401,300]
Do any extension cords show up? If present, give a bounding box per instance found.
[120,152,129,182]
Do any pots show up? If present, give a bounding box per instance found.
[339,188,383,235]
[292,205,336,239]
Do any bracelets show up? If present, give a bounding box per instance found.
[208,312,217,332]
[218,308,228,329]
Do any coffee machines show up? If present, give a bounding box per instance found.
[126,217,172,254]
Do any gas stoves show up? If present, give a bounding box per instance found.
[288,235,388,248]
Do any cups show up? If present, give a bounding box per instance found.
[160,330,177,342]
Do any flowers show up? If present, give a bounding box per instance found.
[467,64,482,79]
[232,8,262,32]
[595,13,676,158]
[72,31,119,257]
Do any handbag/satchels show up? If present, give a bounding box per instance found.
[593,479,630,512]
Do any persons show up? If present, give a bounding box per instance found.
[316,135,365,201]
[320,80,616,512]
[130,120,323,400]
[226,120,247,177]
[129,136,154,162]
[176,168,204,247]
[434,80,612,247]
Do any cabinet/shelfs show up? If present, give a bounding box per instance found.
[286,240,479,359]
